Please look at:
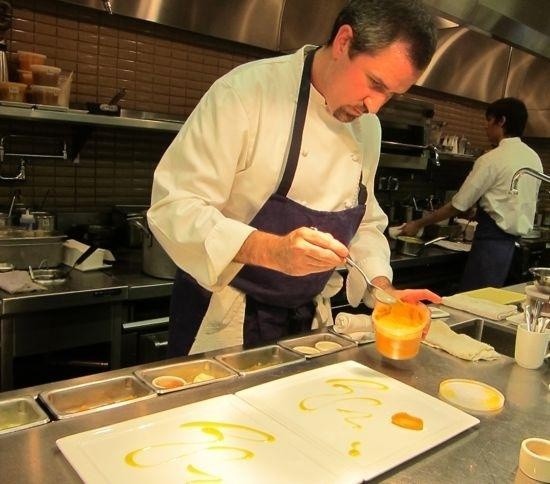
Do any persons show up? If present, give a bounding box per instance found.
[144,0,444,363]
[395,96,545,295]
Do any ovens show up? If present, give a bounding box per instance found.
[379,93,436,171]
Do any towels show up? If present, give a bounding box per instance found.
[434,240,472,251]
[422,320,501,362]
[0,270,48,294]
[188,286,246,356]
[460,287,526,305]
[442,294,517,321]
[333,312,376,344]
[389,223,424,239]
[311,272,344,330]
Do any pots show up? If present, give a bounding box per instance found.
[396,234,449,258]
[127,206,179,282]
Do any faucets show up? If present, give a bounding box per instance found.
[424,194,434,210]
[508,166,548,198]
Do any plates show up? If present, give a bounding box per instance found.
[234,359,481,483]
[56,393,367,483]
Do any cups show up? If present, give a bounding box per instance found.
[514,322,550,370]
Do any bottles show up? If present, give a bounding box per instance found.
[18,209,36,230]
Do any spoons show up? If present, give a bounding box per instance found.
[311,226,400,306]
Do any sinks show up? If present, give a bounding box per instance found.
[447,315,520,361]
